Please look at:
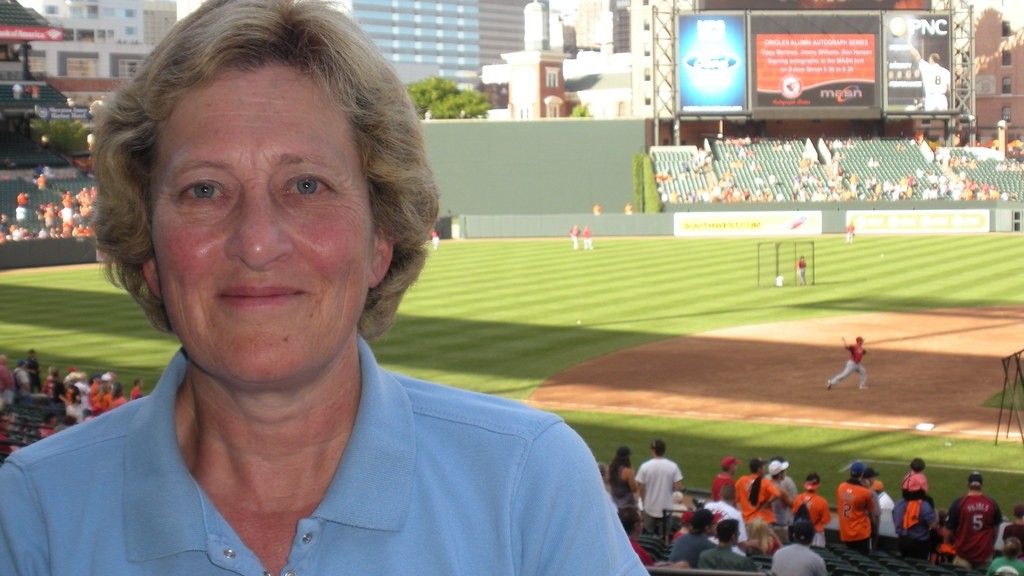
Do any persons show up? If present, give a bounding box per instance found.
[592,203,603,215]
[0,2,651,576]
[624,202,633,215]
[424,110,433,120]
[597,438,1024,576]
[67,98,75,106]
[583,226,592,250]
[826,336,870,390]
[430,227,440,251]
[458,110,470,119]
[0,155,97,243]
[87,133,96,152]
[907,36,951,111]
[847,223,855,244]
[797,256,807,286]
[570,225,580,250]
[0,349,145,459]
[654,131,1024,203]
[12,82,40,101]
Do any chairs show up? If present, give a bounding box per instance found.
[644,136,1024,207]
[0,390,82,462]
[0,173,110,269]
[619,506,985,576]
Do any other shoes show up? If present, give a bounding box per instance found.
[859,385,869,391]
[826,379,831,390]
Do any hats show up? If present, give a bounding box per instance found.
[748,458,767,473]
[802,473,820,491]
[721,456,741,469]
[863,467,880,479]
[690,508,721,530]
[767,460,790,477]
[968,471,983,485]
[16,359,28,367]
[856,337,864,343]
[849,460,868,475]
